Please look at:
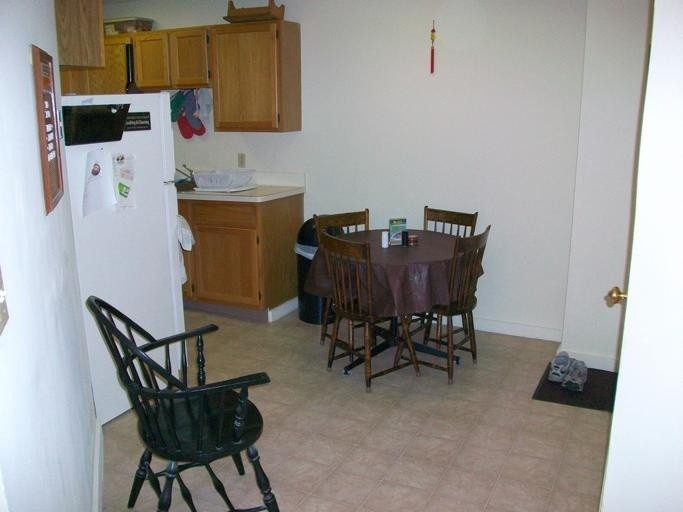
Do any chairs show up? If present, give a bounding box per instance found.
[394,225,491,384]
[319,229,421,393]
[85,296,279,512]
[313,208,400,364]
[408,206,479,351]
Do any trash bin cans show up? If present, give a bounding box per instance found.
[294,216,343,324]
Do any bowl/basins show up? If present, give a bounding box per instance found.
[193,168,255,188]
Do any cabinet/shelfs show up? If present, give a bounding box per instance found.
[133,25,213,90]
[60,34,131,95]
[178,193,304,311]
[211,20,301,132]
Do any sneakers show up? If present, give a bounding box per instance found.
[547,352,587,392]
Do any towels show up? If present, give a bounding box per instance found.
[178,214,196,286]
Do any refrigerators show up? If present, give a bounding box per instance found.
[58,90,189,427]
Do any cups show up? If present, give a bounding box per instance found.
[408,234,416,245]
[380,231,388,248]
[401,230,407,246]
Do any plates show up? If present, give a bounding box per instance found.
[192,187,253,193]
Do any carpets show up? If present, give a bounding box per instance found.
[531,362,617,412]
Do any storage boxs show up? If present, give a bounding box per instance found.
[103,17,153,34]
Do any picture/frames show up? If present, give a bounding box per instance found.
[31,44,64,215]
